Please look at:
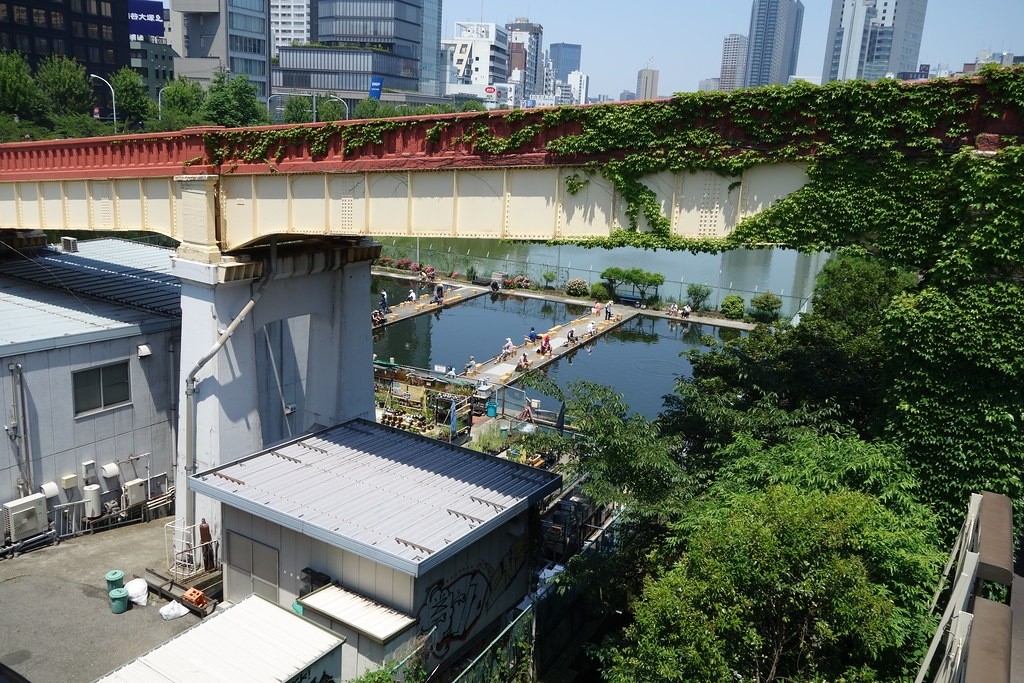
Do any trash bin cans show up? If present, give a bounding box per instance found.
[486,401,499,417]
[104,569,125,594]
[108,588,129,614]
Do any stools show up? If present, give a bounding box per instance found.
[387,287,462,322]
[465,310,623,383]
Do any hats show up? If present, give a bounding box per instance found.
[438,284,443,287]
[609,301,613,305]
[373,310,379,312]
[506,338,511,341]
[409,289,413,292]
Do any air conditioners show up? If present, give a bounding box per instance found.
[3,492,48,543]
[60,236,78,253]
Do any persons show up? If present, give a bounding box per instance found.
[406,290,416,302]
[767,324,775,334]
[503,338,512,356]
[491,280,499,292]
[605,301,613,320]
[566,328,575,343]
[464,356,475,376]
[681,304,691,318]
[524,327,536,346]
[380,289,387,313]
[669,303,678,316]
[426,276,432,286]
[419,272,426,281]
[518,353,529,371]
[589,301,600,315]
[371,309,384,327]
[435,295,443,306]
[541,337,551,355]
[445,367,455,378]
[436,284,443,298]
[588,322,597,336]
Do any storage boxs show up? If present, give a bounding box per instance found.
[182,587,207,606]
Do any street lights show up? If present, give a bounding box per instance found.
[91,74,116,133]
[330,95,348,120]
[267,95,279,115]
[323,99,338,110]
[159,87,171,120]
